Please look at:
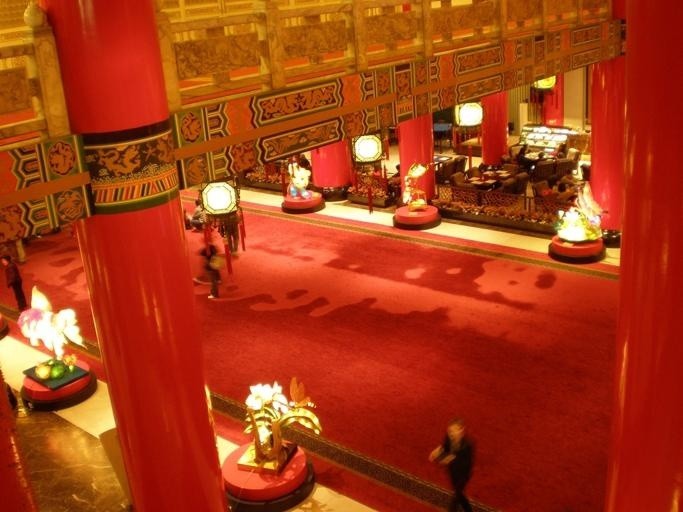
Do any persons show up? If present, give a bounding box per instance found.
[428,417,475,512]
[0,253,27,310]
[300,142,585,201]
[185,198,226,299]
